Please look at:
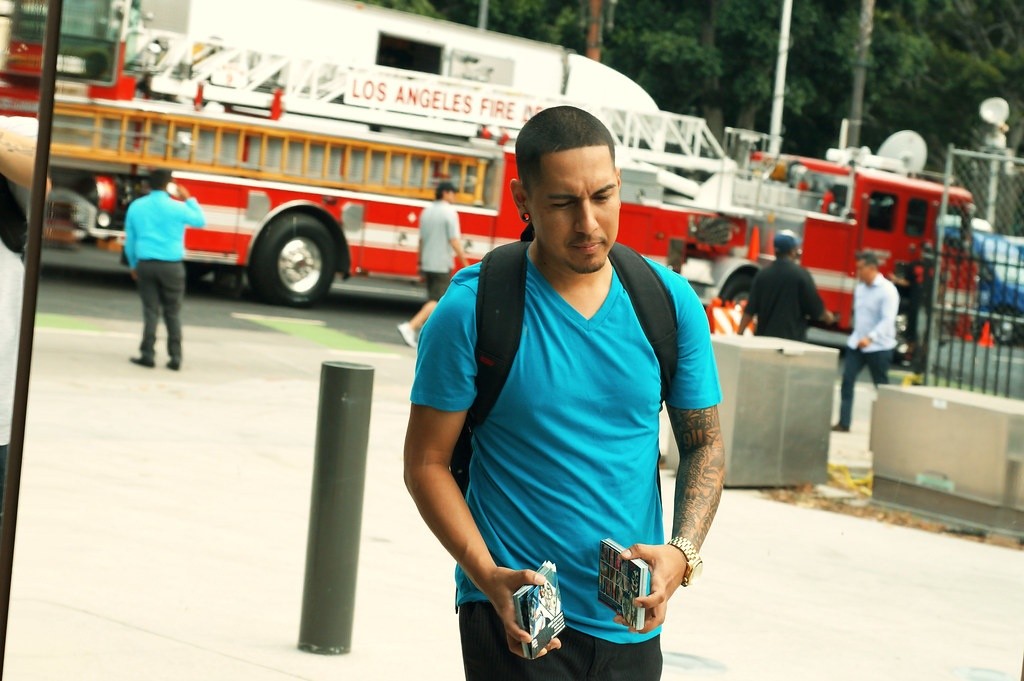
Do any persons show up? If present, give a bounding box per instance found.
[403,107,725,681]
[398,181,470,348]
[831,251,900,433]
[125,169,205,370]
[736,229,834,344]
[889,240,943,361]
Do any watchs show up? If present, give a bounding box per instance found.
[669,537,704,588]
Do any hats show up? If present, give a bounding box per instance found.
[774,230,803,249]
[438,182,459,193]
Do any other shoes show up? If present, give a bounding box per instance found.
[831,423,850,433]
[130,356,155,368]
[398,321,417,348]
[168,360,179,370]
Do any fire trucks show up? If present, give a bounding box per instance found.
[0,0,978,338]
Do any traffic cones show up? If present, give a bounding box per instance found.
[976,320,994,347]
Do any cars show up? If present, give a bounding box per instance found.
[936,227,1024,347]
